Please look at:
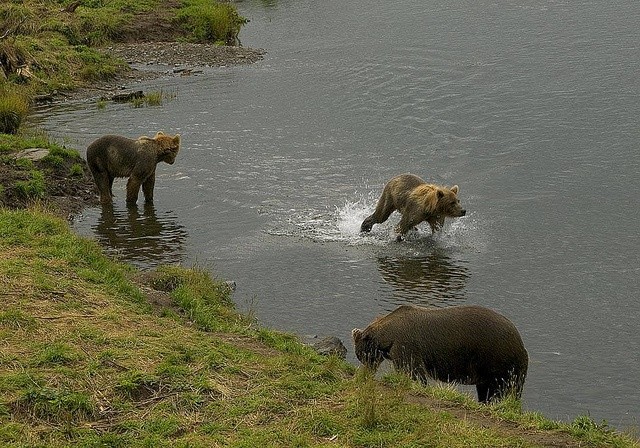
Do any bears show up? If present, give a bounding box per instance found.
[352,304,529,406]
[86,131,181,202]
[360,173,467,243]
